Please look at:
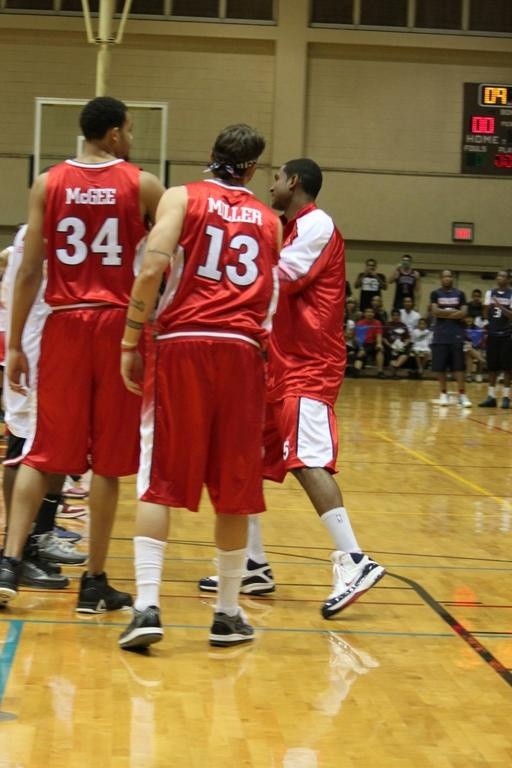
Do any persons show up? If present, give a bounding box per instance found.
[118,121,285,645]
[479,270,512,408]
[430,269,473,408]
[0,222,90,588]
[344,254,511,384]
[199,158,386,619]
[0,97,167,614]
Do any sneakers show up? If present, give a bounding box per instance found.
[199,557,276,594]
[117,605,165,648]
[439,394,510,408]
[208,608,256,646]
[77,571,133,613]
[345,362,423,384]
[321,550,386,617]
[0,557,22,607]
[448,371,483,382]
[0,472,91,589]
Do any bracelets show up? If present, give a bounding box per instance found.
[121,347,137,352]
[121,339,138,345]
